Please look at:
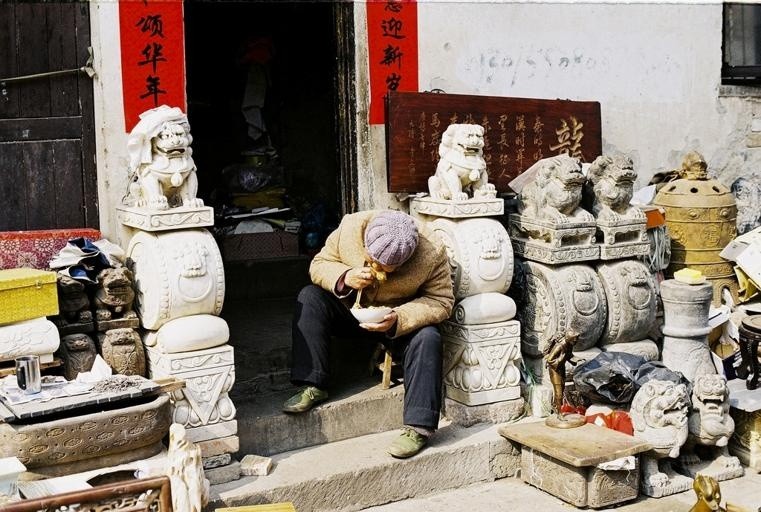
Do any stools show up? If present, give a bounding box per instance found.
[367,337,404,390]
[739,315,761,390]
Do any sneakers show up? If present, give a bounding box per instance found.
[283,385,331,413]
[386,427,429,459]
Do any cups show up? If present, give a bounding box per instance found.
[15,356,42,396]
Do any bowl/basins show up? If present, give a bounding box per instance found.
[350,308,392,326]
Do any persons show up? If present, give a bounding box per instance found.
[544,330,583,419]
[282,205,455,461]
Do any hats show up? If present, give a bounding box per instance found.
[363,211,419,268]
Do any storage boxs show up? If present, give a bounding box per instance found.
[223,232,299,261]
[0,268,59,325]
